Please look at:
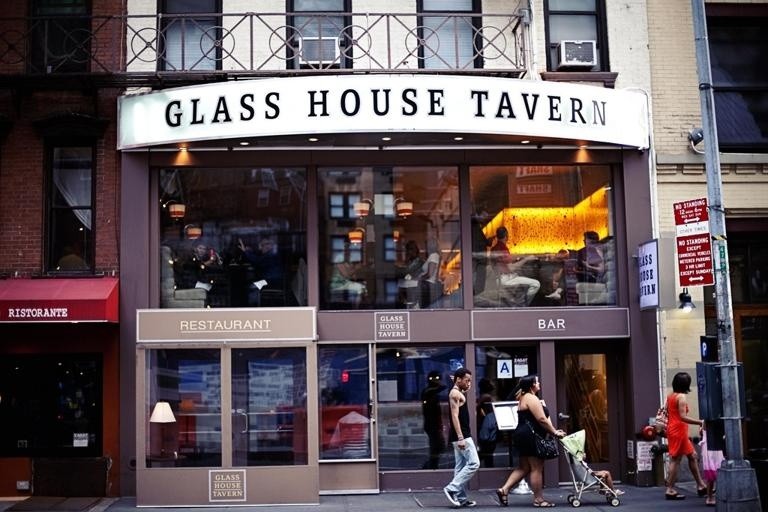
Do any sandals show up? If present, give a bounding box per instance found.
[495,488,508,506]
[532,499,556,507]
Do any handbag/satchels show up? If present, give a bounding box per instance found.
[532,433,560,459]
[654,407,669,438]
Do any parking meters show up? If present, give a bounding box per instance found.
[696,334,746,461]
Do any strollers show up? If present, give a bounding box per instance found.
[554,427,621,505]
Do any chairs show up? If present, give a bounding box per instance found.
[474,261,530,307]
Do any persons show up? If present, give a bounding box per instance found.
[475,377,497,466]
[698,416,725,505]
[419,368,448,470]
[163,219,297,307]
[496,373,567,507]
[56,244,86,267]
[578,452,626,497]
[661,371,716,500]
[328,236,445,310]
[543,229,605,301]
[588,374,608,419]
[442,367,480,508]
[489,225,541,305]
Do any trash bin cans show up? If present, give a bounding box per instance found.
[744,448,768,512]
[621,437,657,486]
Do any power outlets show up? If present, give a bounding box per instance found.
[150,402,176,455]
[679,287,696,311]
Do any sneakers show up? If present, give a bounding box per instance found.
[544,287,564,300]
[459,499,477,508]
[614,488,626,496]
[443,487,460,507]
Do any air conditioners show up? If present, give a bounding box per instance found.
[298,37,340,65]
[556,40,598,68]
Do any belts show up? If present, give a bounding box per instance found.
[499,271,513,276]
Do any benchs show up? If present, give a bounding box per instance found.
[576,241,617,304]
[161,246,208,308]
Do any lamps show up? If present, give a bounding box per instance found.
[352,199,373,219]
[348,227,366,245]
[182,223,202,243]
[164,199,186,221]
[393,197,413,219]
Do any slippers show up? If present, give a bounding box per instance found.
[705,498,716,506]
[697,485,716,498]
[665,491,686,500]
